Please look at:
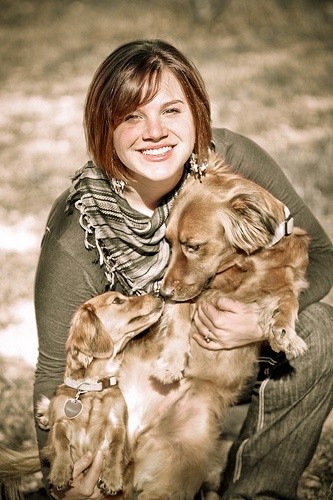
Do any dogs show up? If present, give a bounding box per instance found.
[0,147,313,500]
[33,290,165,500]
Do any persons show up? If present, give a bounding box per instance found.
[34,40,333,500]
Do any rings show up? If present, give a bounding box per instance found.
[204,330,213,342]
